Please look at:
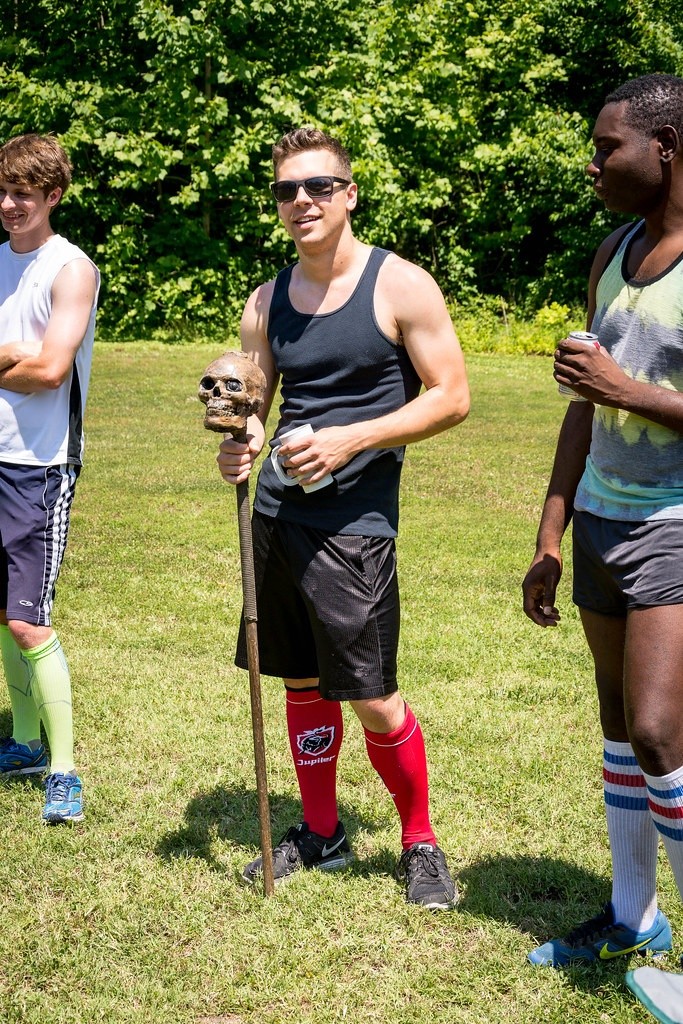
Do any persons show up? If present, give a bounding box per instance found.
[521,70,683,1024]
[0,135,108,826]
[216,129,472,916]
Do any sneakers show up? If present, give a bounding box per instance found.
[528,901,673,969]
[41,772,85,822]
[242,821,355,887]
[0,737,50,776]
[395,842,458,911]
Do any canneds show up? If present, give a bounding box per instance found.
[557,331,599,396]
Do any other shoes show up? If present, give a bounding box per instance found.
[625,967,683,1024]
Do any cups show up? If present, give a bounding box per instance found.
[271,423,334,494]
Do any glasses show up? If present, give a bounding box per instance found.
[271,176,351,203]
